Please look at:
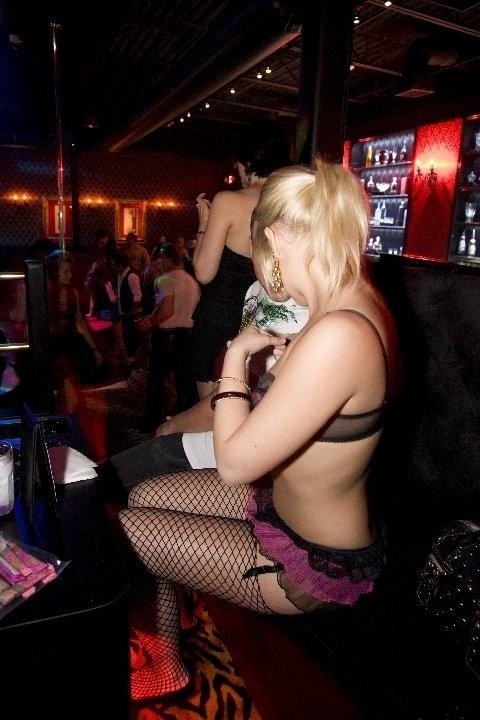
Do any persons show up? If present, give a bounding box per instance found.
[92,206,315,526]
[113,157,398,705]
[1,227,203,435]
[190,116,288,402]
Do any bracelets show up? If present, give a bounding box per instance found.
[195,231,206,236]
[211,376,252,413]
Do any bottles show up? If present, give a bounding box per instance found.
[397,200,405,225]
[466,229,477,256]
[457,228,466,255]
[391,176,398,194]
[367,238,374,251]
[374,201,382,226]
[0,440,15,517]
[380,200,388,221]
[364,145,407,166]
[372,235,383,251]
[366,175,375,190]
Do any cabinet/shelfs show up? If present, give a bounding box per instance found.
[447,118,480,262]
[353,129,414,257]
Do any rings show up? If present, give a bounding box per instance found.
[196,204,199,207]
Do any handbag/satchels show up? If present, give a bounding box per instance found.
[411,509,479,675]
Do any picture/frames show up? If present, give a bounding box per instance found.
[114,199,146,244]
[42,194,73,240]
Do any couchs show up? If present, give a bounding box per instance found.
[195,254,480,720]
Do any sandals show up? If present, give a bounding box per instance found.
[129,673,195,706]
